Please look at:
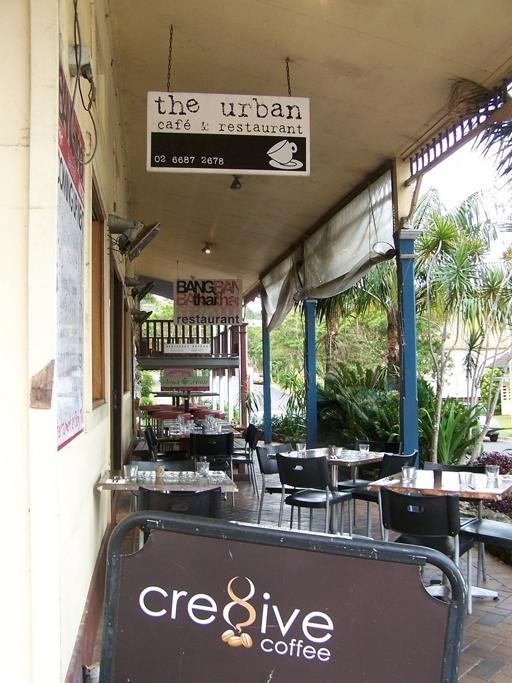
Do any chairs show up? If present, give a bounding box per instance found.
[99,403,512,683]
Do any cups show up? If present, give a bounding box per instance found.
[359,444,370,453]
[124,465,138,479]
[459,472,472,486]
[296,443,307,453]
[486,465,499,479]
[401,466,416,481]
[155,464,165,478]
[196,461,209,477]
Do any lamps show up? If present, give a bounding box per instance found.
[108,214,161,261]
[201,242,215,254]
[231,175,242,188]
